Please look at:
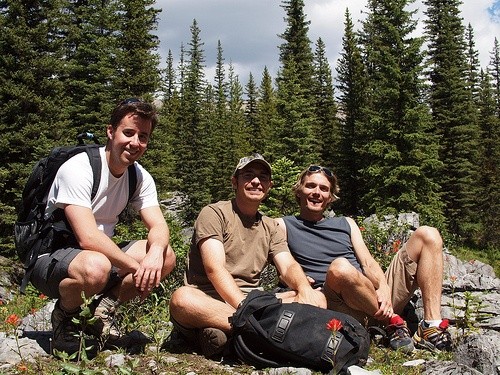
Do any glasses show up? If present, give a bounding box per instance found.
[308,165,333,179]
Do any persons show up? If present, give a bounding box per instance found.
[271,164,451,354]
[29,98,177,362]
[168,152,327,357]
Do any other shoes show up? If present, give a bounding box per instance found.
[170,328,228,358]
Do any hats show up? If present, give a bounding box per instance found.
[229,289,279,327]
[234,153,277,176]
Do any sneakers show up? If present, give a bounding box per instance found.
[386,315,414,353]
[412,320,453,354]
[85,303,131,347]
[50,301,82,354]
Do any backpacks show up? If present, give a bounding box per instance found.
[225,301,388,374]
[14,145,137,299]
